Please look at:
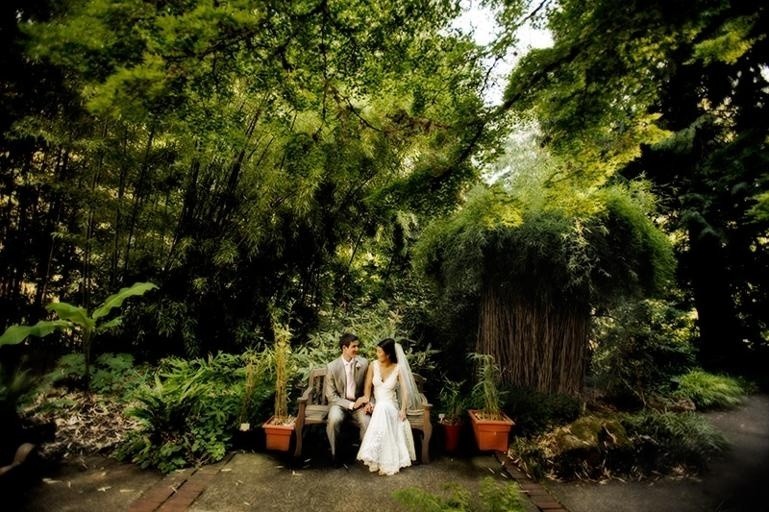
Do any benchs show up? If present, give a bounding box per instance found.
[292,366,434,466]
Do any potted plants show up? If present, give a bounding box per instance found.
[262,323,298,455]
[437,351,515,460]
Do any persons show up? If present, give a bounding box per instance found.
[353,336,412,477]
[323,333,371,459]
